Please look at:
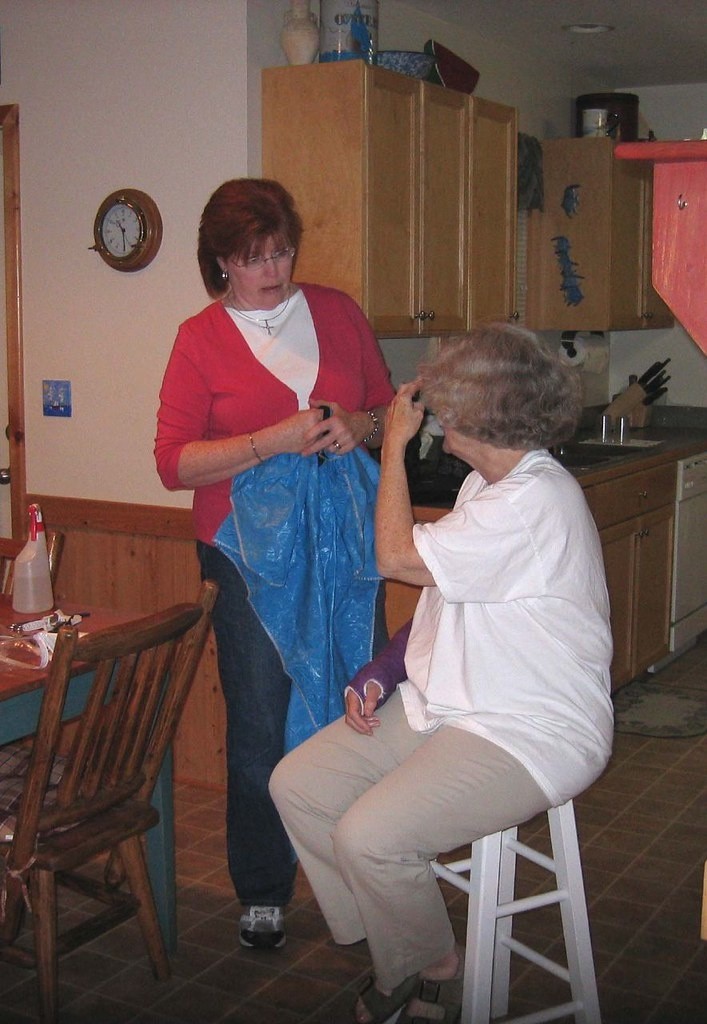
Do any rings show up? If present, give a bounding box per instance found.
[333,441,341,449]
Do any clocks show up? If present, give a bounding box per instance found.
[92,184,162,272]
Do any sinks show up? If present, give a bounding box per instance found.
[561,443,647,457]
[546,453,616,470]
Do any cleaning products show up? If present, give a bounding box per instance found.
[12,503,55,614]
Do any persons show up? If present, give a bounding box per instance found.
[153,177,397,951]
[266,319,615,1024]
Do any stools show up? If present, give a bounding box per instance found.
[428,799,601,1024]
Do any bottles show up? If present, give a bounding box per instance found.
[12,503,54,614]
[616,415,631,445]
[279,0,320,65]
[598,415,611,445]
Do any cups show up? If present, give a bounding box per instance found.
[583,110,620,138]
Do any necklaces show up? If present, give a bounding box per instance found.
[227,289,291,336]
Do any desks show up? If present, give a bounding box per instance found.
[0,584,180,947]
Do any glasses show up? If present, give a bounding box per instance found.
[227,246,294,270]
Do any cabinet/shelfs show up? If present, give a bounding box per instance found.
[529,136,678,333]
[251,61,470,339]
[581,466,681,694]
[465,95,517,341]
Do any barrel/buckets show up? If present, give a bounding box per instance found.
[320,0,379,67]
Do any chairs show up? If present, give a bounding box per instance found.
[0,527,65,599]
[0,579,223,1024]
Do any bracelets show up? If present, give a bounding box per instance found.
[249,432,264,464]
[363,409,380,443]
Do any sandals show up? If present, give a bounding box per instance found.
[352,948,466,1024]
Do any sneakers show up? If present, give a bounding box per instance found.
[239,903,288,948]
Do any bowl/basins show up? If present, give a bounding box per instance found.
[576,93,639,142]
[379,51,436,81]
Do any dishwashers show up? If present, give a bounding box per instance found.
[669,452,707,652]
